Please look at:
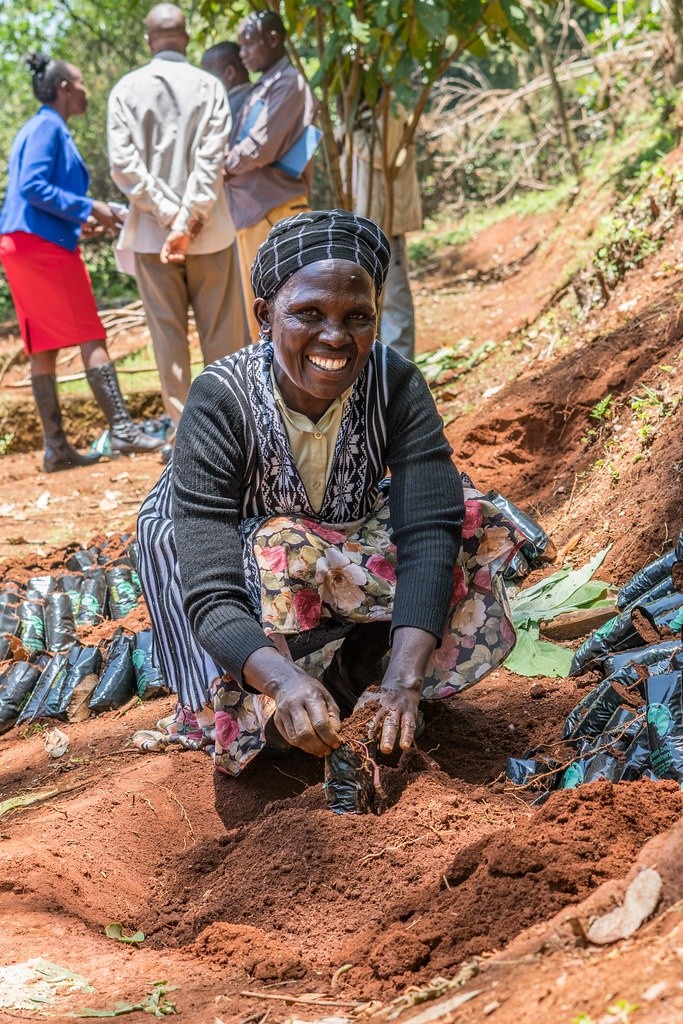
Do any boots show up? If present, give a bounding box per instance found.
[28,362,165,473]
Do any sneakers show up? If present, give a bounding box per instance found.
[322,647,426,744]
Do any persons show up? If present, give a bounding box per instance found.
[135,210,516,777]
[0,55,166,472]
[335,65,422,363]
[199,42,281,348]
[222,11,323,344]
[106,3,252,461]
